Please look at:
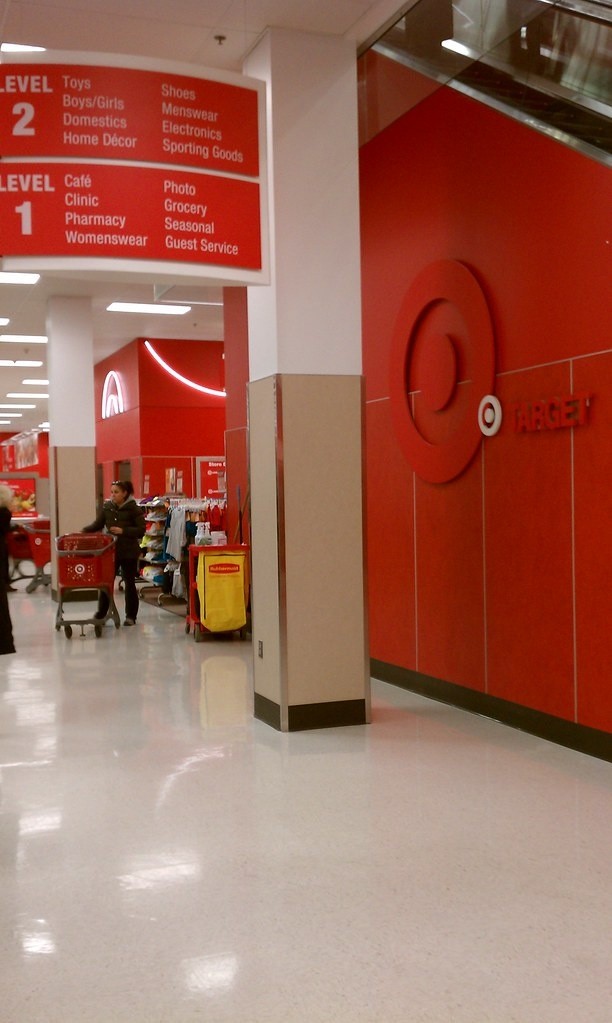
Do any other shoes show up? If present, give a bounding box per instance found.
[124,618,136,626]
[6,585,18,592]
[0,644,16,655]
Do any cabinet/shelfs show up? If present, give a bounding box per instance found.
[135,498,170,564]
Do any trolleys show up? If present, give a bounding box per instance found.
[53,532,121,637]
[9,521,51,593]
[185,542,250,641]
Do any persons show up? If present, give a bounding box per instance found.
[80,480,147,625]
[0,483,20,655]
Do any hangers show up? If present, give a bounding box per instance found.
[167,498,227,513]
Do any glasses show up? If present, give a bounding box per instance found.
[112,481,119,485]
